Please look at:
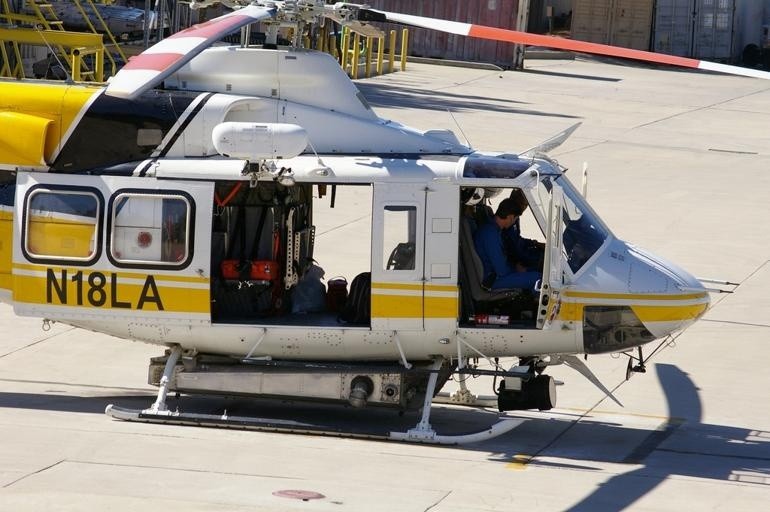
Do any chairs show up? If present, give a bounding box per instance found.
[461,205,523,302]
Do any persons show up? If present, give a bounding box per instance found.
[505,188,545,268]
[474,197,542,322]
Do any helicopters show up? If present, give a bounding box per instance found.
[1,1,770,446]
